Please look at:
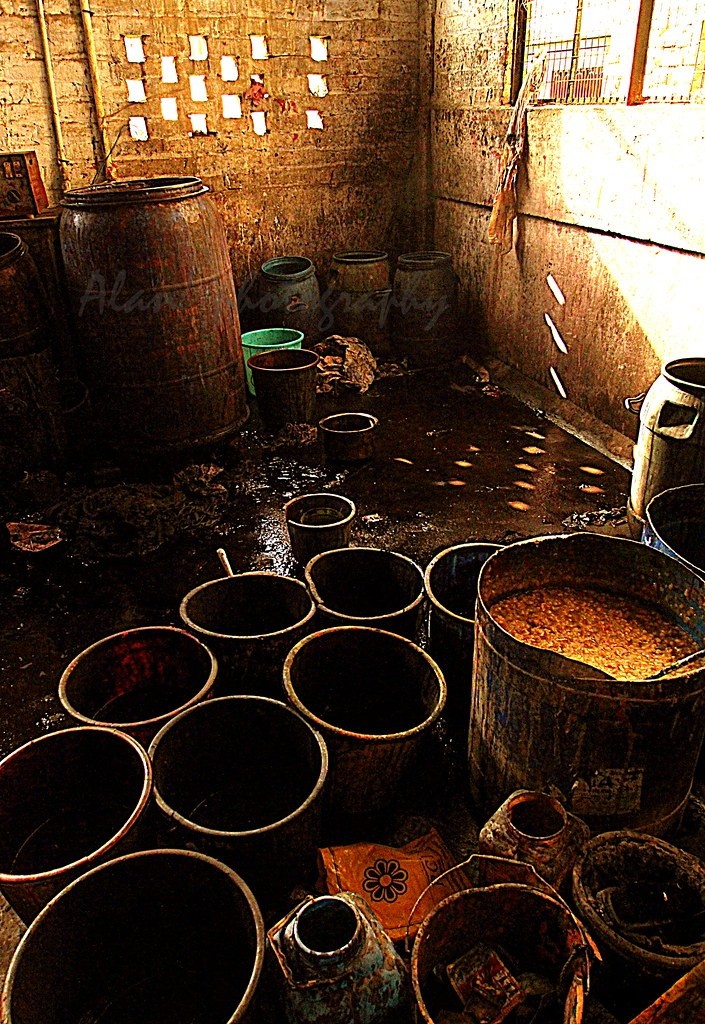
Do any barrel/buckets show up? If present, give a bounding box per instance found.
[576,829,705,993]
[399,848,594,1024]
[285,350,705,842]
[4,569,330,1022]
[0,166,460,463]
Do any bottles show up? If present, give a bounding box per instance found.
[270,889,406,1024]
[472,792,585,896]
